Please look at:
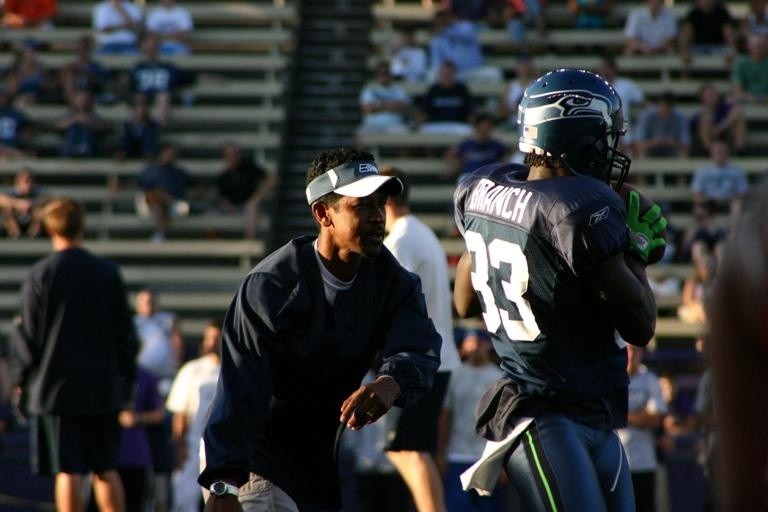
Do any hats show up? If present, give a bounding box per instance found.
[306,159,404,204]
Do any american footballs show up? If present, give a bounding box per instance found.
[610,180,667,265]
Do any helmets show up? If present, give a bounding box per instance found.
[518,67,630,185]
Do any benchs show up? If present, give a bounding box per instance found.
[2,0,303,380]
[359,0,767,356]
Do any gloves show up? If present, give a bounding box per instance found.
[627,191,668,258]
